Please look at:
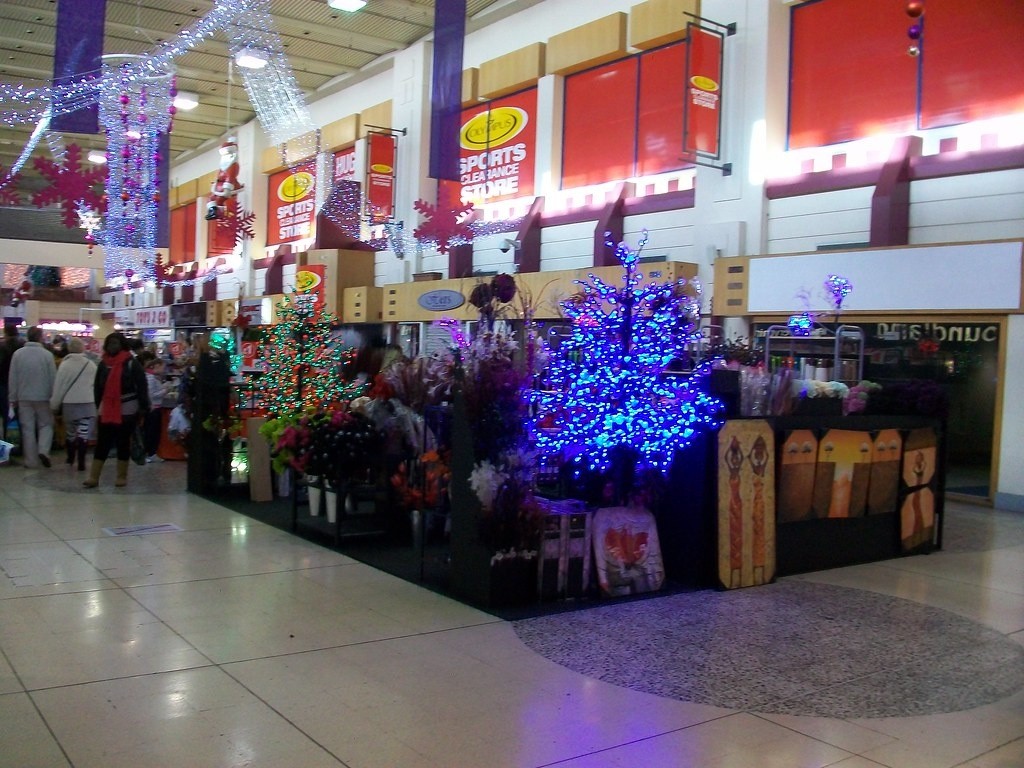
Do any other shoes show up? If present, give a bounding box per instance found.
[24,464,36,469]
[39,450,51,468]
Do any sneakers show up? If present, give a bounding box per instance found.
[145,454,165,463]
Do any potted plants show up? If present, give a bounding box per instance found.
[172,349,233,495]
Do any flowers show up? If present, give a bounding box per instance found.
[275,411,357,473]
[391,448,452,511]
[257,407,333,477]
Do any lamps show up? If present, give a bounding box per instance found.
[88,150,107,164]
[173,91,199,111]
[235,47,269,69]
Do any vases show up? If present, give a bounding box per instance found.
[411,510,430,565]
[324,479,354,524]
[305,475,327,517]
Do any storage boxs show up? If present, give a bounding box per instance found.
[381,261,699,322]
[307,249,375,323]
[221,293,291,325]
[713,238,1024,318]
[343,285,383,324]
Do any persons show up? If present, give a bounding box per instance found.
[0,323,410,487]
[205,140,244,221]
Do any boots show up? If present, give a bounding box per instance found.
[76,437,87,471]
[114,460,128,486]
[83,459,103,487]
[66,439,77,465]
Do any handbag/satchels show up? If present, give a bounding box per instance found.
[130,425,145,465]
[54,403,63,426]
